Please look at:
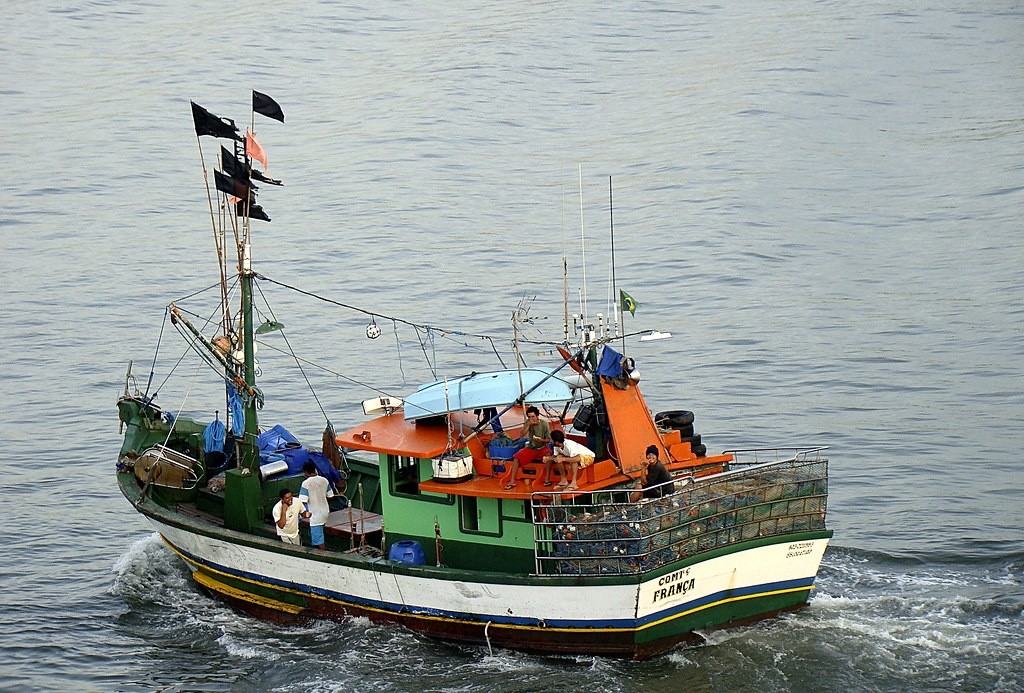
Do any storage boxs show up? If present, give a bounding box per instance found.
[489,439,526,472]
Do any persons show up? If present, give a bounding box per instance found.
[543,430,595,490]
[298,458,335,550]
[630,445,675,503]
[272,489,312,545]
[504,406,553,490]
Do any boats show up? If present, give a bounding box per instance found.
[403,366,574,422]
[110,84,837,664]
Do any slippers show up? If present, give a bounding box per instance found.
[564,485,578,493]
[504,483,516,489]
[543,480,552,487]
[553,483,570,490]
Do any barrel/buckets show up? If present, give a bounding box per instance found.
[388,540,426,565]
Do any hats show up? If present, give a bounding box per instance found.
[645,444,659,458]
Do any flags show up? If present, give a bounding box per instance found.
[596,346,624,378]
[621,291,641,317]
[234,141,250,166]
[221,145,259,191]
[557,346,586,378]
[214,170,258,204]
[234,200,271,222]
[191,100,243,142]
[252,90,284,123]
[246,132,267,169]
[251,169,284,186]
